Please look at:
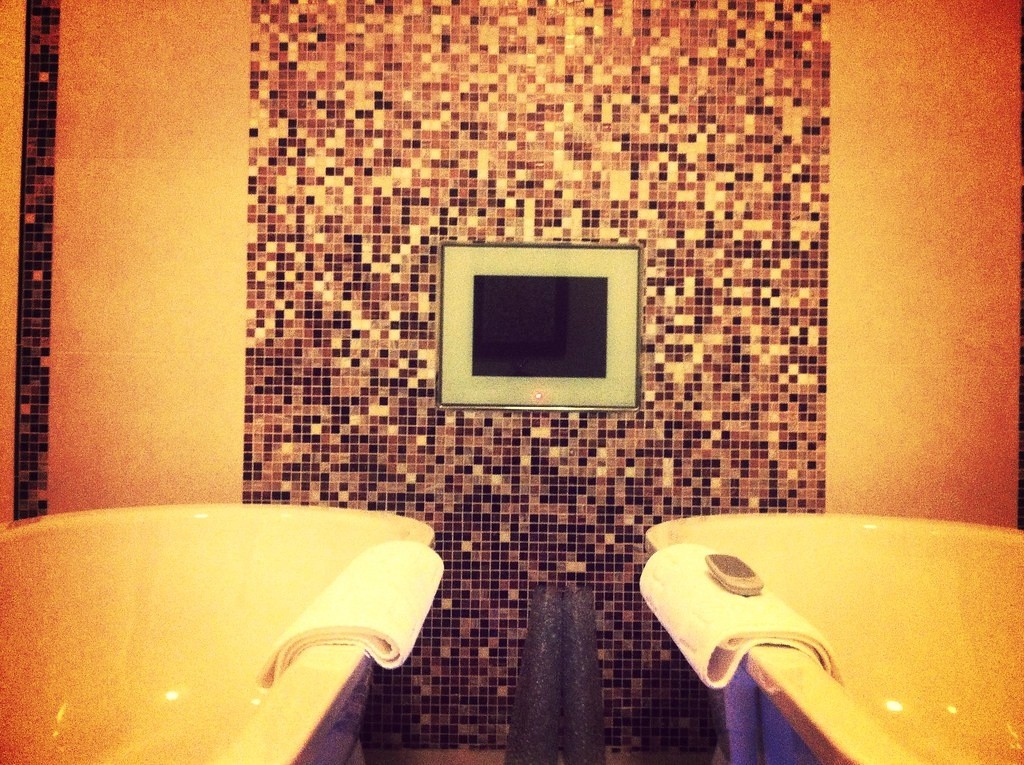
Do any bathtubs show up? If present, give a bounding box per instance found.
[641,513,1024,765]
[0,503,435,765]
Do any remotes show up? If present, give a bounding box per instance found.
[706,553,764,596]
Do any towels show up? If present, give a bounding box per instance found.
[638,543,845,765]
[252,543,445,694]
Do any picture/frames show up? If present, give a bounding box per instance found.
[437,242,642,413]
